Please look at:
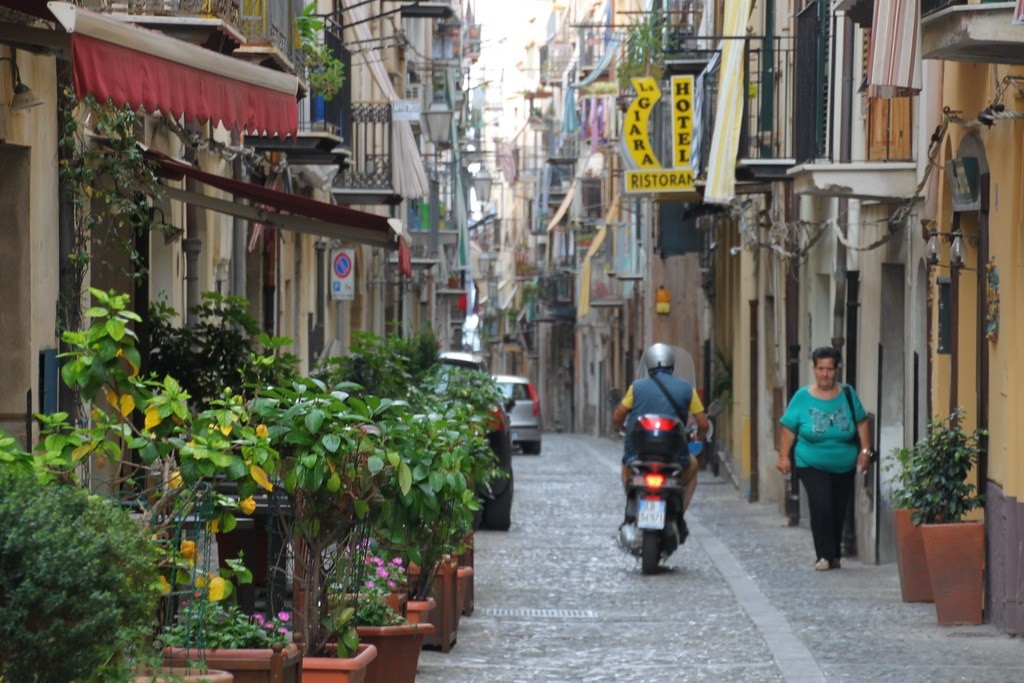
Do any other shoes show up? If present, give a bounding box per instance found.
[832,557,840,567]
[815,558,829,571]
[681,521,688,535]
[619,514,635,530]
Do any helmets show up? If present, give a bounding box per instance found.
[645,343,674,369]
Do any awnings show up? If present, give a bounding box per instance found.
[124,153,417,280]
[1,0,299,142]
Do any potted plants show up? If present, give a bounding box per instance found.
[880,404,989,626]
[1,287,511,683]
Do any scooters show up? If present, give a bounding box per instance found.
[606,344,725,574]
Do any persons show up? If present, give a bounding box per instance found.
[612,342,709,546]
[776,346,873,572]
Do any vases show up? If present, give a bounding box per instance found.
[161,645,302,682]
[320,623,435,683]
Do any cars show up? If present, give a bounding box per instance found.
[426,352,517,530]
[489,374,543,455]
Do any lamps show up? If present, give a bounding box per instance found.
[146,207,188,246]
[473,164,493,201]
[0,57,46,113]
[366,101,454,180]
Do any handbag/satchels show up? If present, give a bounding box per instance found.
[843,384,878,463]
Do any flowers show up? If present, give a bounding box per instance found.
[158,599,292,655]
[341,539,408,625]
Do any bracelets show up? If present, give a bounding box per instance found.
[861,448,873,457]
